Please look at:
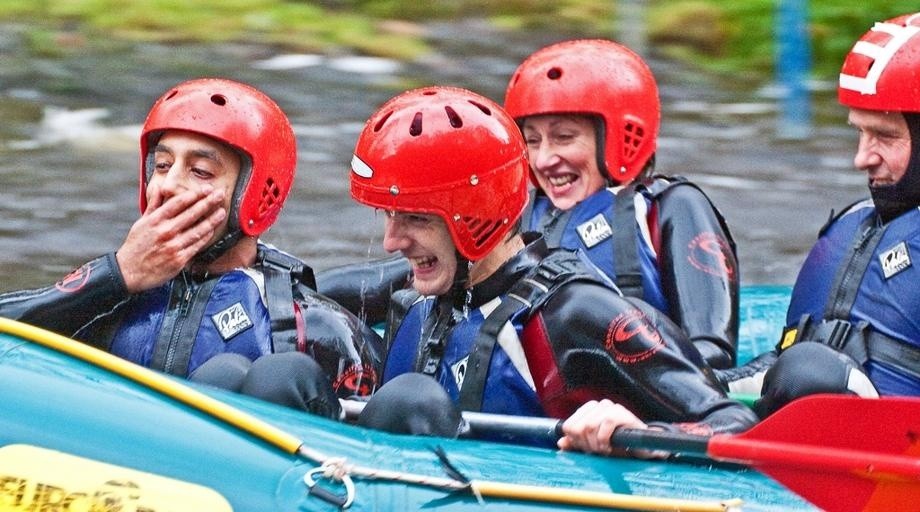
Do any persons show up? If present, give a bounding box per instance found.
[711,11,919,415]
[502,36,742,381]
[0,77,377,429]
[256,81,762,466]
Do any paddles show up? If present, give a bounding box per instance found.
[336,394,920,511]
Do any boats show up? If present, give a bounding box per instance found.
[0,278,851,512]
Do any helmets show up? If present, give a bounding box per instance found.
[504,36,660,184]
[135,76,297,237]
[837,12,920,115]
[346,85,533,262]
